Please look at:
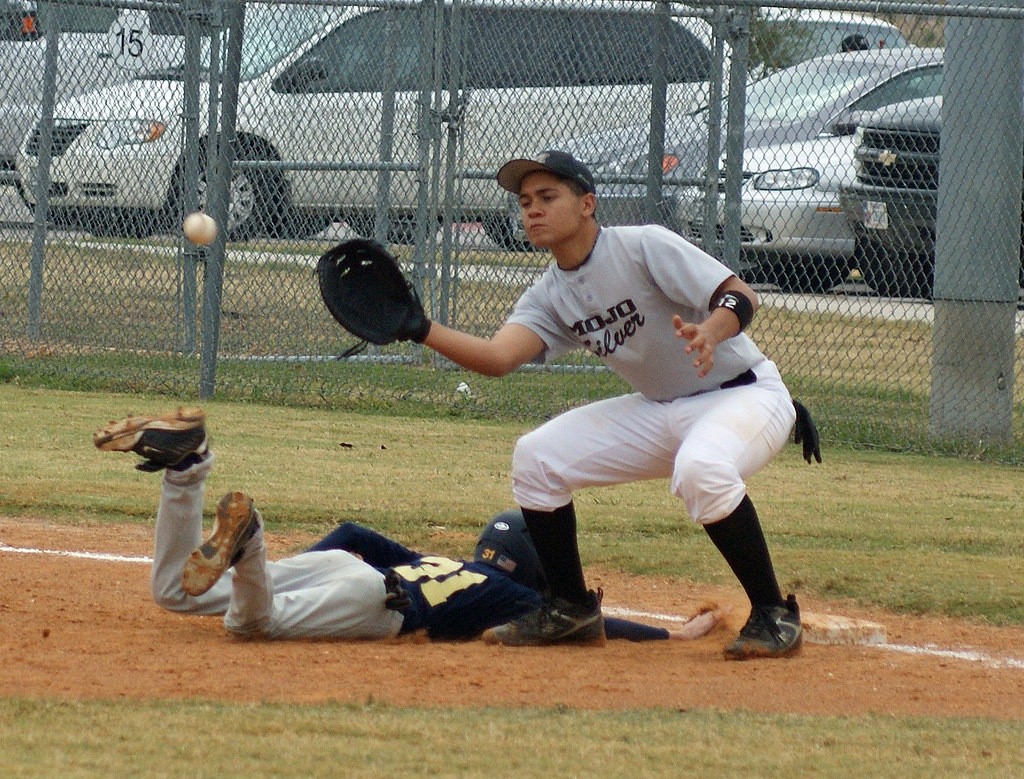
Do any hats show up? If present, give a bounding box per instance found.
[497,150,596,195]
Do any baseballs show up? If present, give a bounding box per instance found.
[183,212,217,244]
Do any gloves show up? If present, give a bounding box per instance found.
[385,571,414,610]
[792,397,822,466]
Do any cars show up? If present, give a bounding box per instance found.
[0,2,1024,297]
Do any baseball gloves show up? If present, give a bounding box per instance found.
[311,236,432,362]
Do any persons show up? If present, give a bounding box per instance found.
[94,407,725,642]
[841,34,869,52]
[318,150,803,661]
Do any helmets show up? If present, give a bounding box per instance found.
[475,508,550,589]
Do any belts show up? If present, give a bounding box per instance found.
[720,369,757,390]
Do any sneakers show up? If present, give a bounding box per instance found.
[94,405,208,473]
[725,592,801,662]
[481,586,606,647]
[181,492,260,597]
